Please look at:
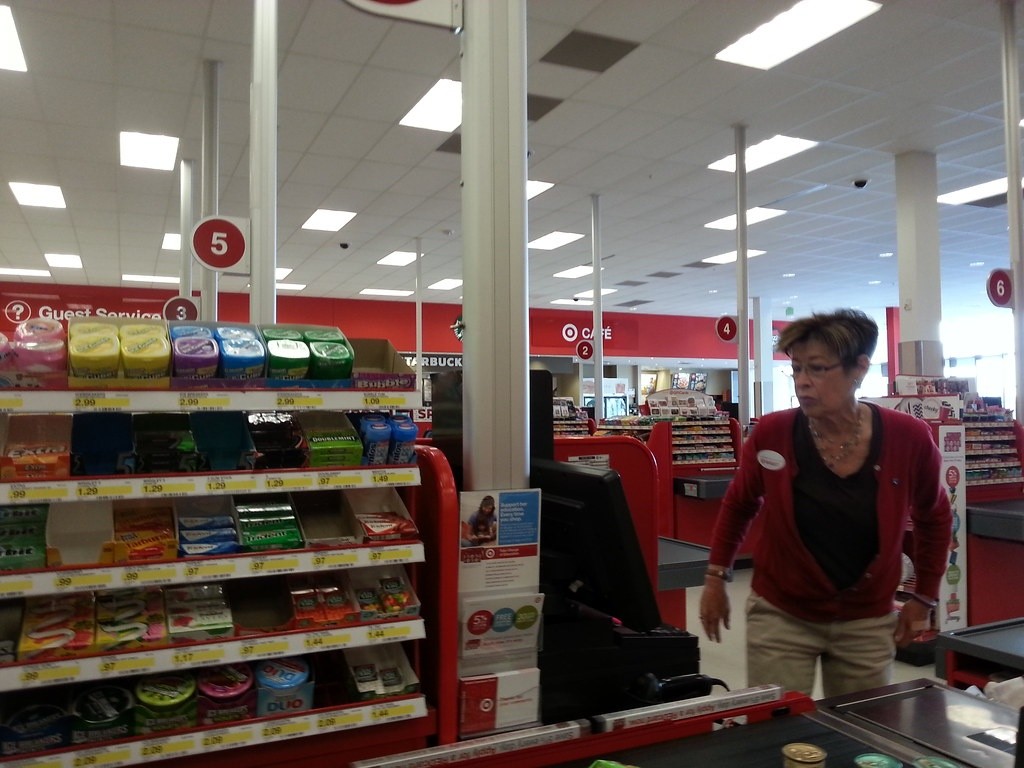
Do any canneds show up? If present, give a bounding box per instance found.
[782,742,827,768]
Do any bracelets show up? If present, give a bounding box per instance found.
[705,567,732,583]
[912,593,938,608]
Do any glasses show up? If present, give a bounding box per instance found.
[781,359,842,377]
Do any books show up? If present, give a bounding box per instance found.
[459,488,540,622]
[461,592,545,656]
[459,667,541,735]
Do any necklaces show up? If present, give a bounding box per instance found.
[807,403,864,469]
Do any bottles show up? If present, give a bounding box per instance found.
[916,380,936,394]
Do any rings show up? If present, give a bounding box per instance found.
[699,616,705,619]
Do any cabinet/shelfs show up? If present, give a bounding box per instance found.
[553,416,759,631]
[0,389,429,768]
[964,420,1024,627]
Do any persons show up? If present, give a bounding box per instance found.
[460,495,498,546]
[700,306,954,702]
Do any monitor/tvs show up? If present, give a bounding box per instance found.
[530,456,663,634]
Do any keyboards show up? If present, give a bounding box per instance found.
[637,625,689,638]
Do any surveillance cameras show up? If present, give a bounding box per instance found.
[573,298,578,301]
[340,244,349,249]
[851,177,871,188]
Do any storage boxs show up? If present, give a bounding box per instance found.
[69,406,135,477]
[131,413,199,475]
[95,588,171,656]
[341,486,419,544]
[341,643,421,702]
[18,595,96,662]
[1,412,74,482]
[302,413,365,469]
[344,569,422,624]
[221,578,297,637]
[347,336,417,391]
[192,416,258,472]
[287,490,363,548]
[166,582,233,645]
[45,502,114,569]
